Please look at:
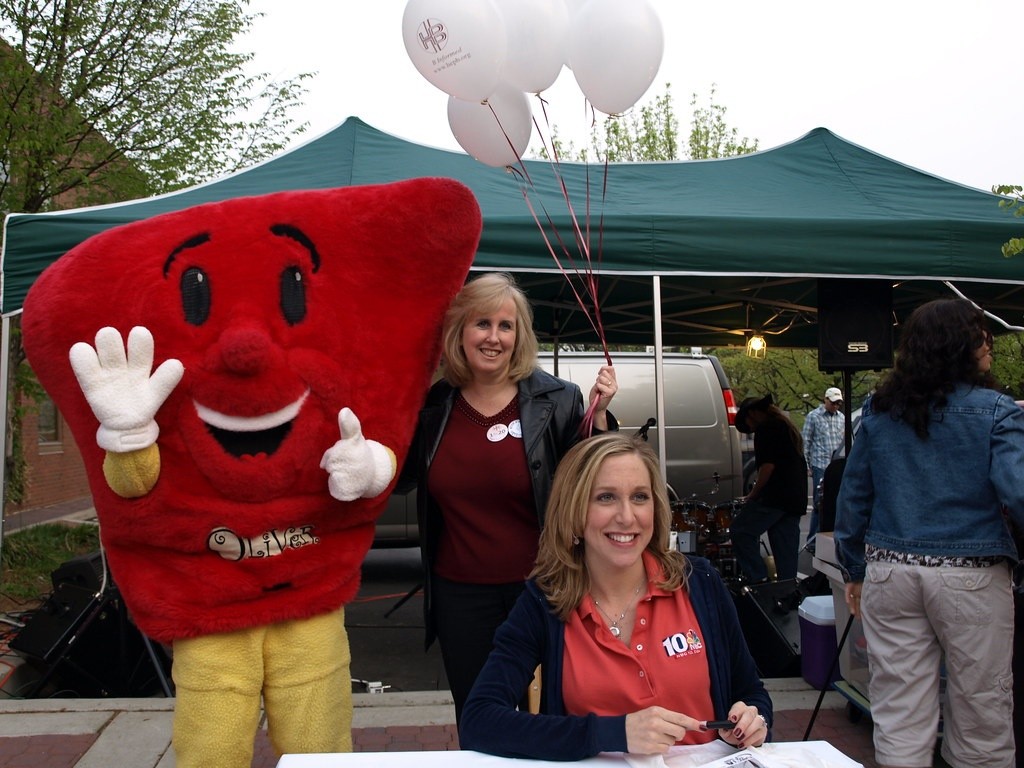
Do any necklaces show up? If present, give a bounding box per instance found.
[590,574,647,636]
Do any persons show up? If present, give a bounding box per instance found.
[460,432,775,762]
[730,396,807,588]
[803,389,845,550]
[389,273,644,735]
[833,298,1024,767]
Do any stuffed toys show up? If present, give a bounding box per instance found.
[23,176,483,768]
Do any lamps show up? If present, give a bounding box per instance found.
[747,329,766,359]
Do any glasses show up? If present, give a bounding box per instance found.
[828,400,841,405]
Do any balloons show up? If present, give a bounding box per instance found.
[402,0,682,172]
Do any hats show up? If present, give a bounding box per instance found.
[734,394,772,434]
[826,388,843,402]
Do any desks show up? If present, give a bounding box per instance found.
[276,740,865,768]
[813,532,871,721]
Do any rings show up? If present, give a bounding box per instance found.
[607,381,611,386]
[851,593,854,598]
[759,715,765,723]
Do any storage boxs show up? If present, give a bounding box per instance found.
[798,595,840,691]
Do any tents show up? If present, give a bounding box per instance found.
[1,118,1023,421]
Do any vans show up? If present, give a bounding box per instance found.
[366,350,748,547]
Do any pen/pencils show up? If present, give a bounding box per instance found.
[700,721,736,729]
